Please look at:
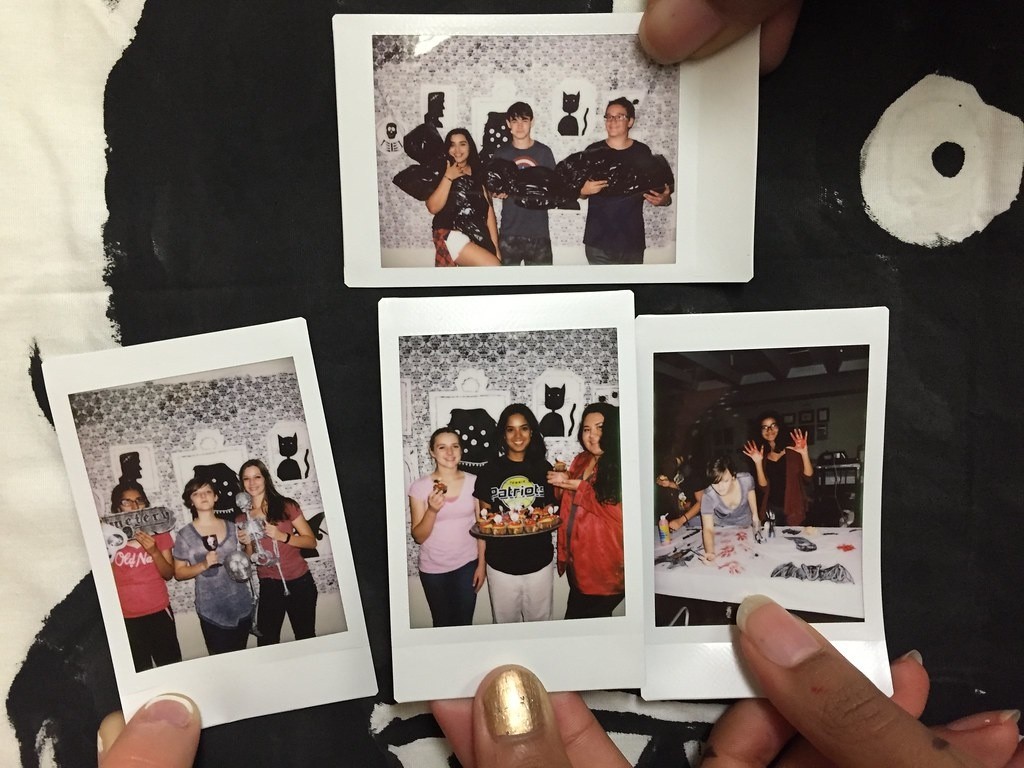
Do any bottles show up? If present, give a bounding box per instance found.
[658,512,672,545]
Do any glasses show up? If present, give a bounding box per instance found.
[760,423,778,431]
[604,114,630,122]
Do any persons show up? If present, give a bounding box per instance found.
[95,691,203,767]
[109,482,183,672]
[425,127,503,266]
[698,456,759,559]
[544,403,625,619]
[431,663,636,768]
[579,97,673,264]
[489,102,557,266]
[172,477,257,656]
[700,593,1024,768]
[410,426,486,627]
[234,457,337,648]
[473,402,560,625]
[636,0,804,72]
[656,445,704,530]
[743,408,816,527]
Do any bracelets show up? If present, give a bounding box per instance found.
[656,196,671,206]
[684,514,688,521]
[282,532,291,544]
[444,175,452,184]
